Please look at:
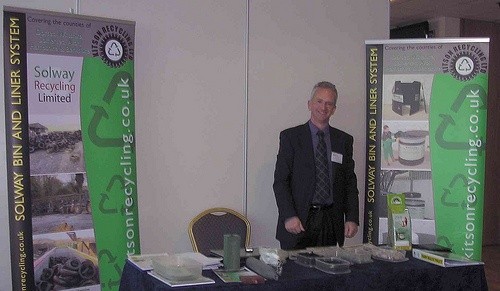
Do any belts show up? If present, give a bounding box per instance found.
[310,206,335,214]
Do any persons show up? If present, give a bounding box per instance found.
[272,81,360,251]
[382,124,399,167]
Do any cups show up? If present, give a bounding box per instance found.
[224,234,241,269]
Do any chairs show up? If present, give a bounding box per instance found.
[187,207,251,254]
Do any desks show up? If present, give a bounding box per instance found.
[120,244,488,291]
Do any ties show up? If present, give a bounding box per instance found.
[314,131,331,204]
